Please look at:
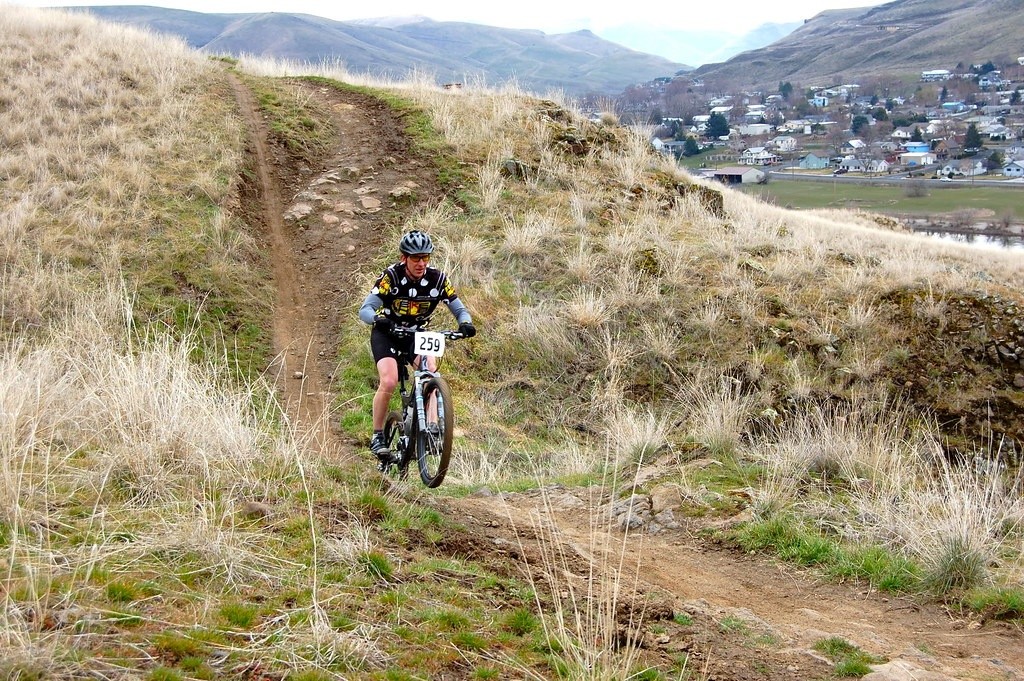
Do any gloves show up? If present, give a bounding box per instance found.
[376,317,395,335]
[458,323,476,340]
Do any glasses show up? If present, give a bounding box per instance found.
[407,253,430,263]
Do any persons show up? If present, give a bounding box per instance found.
[358,230,476,455]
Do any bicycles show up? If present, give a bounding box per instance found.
[364,312,468,491]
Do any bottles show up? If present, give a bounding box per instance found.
[406,400,414,419]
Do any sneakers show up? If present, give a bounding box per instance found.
[424,421,443,454]
[368,432,391,460]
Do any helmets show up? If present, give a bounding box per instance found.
[399,230,435,256]
